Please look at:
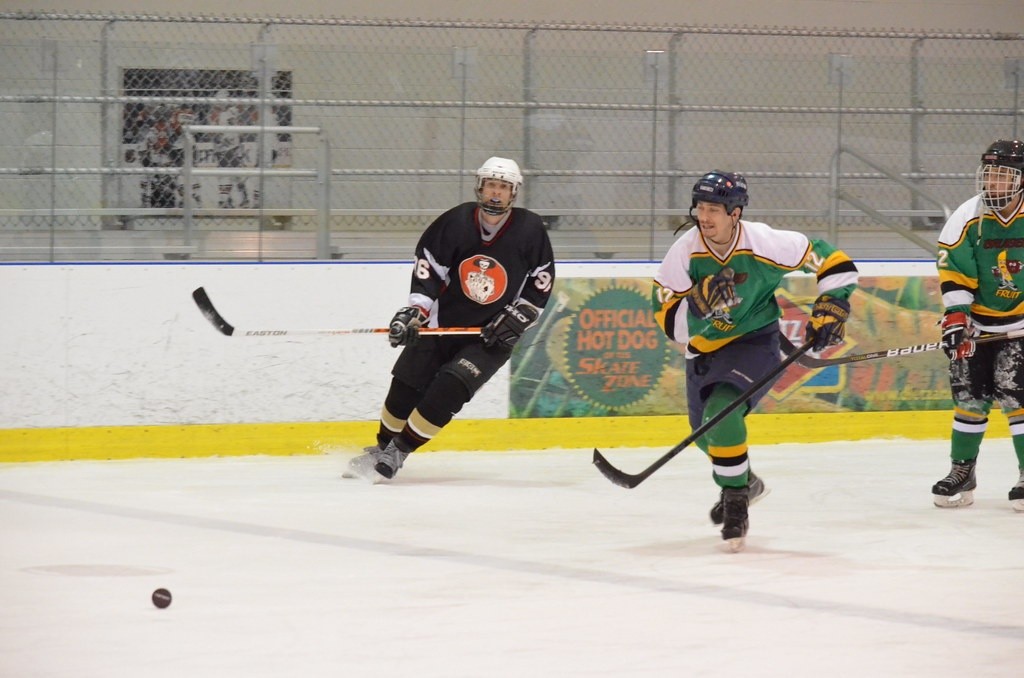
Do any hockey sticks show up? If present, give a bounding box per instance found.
[778,328,1024,370]
[591,336,819,490]
[190,285,482,338]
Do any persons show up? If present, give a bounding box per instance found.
[134,89,280,209]
[343,157,557,482]
[932,138,1024,511]
[652,168,858,551]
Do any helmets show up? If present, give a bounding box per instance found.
[474,157,524,216]
[692,169,749,216]
[976,139,1024,212]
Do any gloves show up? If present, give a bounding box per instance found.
[480,304,539,354]
[389,307,430,348]
[805,294,850,352]
[686,267,744,325]
[938,312,976,360]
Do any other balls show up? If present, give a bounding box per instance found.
[152,588,172,609]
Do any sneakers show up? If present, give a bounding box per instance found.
[367,435,415,486]
[711,470,771,527]
[1007,465,1024,513]
[342,433,389,478]
[721,485,751,552]
[932,458,977,507]
